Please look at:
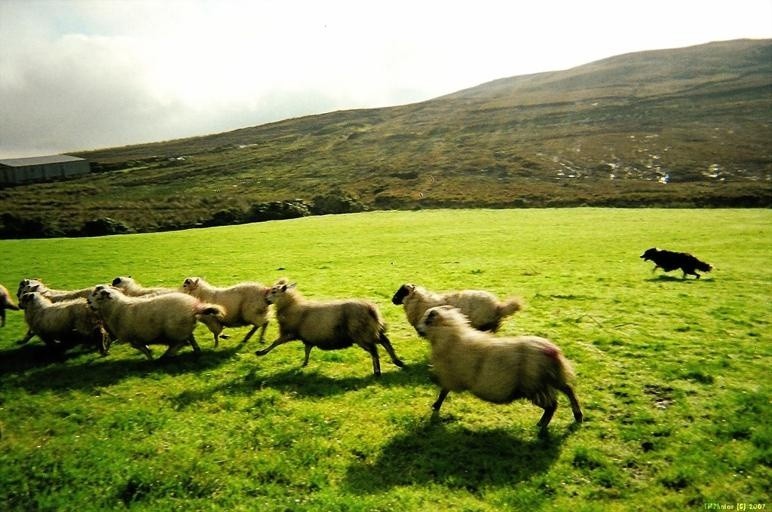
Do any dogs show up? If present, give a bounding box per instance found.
[640,248,712,280]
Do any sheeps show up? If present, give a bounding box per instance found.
[392,283,521,333]
[0,276,270,364]
[256,279,404,379]
[415,304,584,426]
